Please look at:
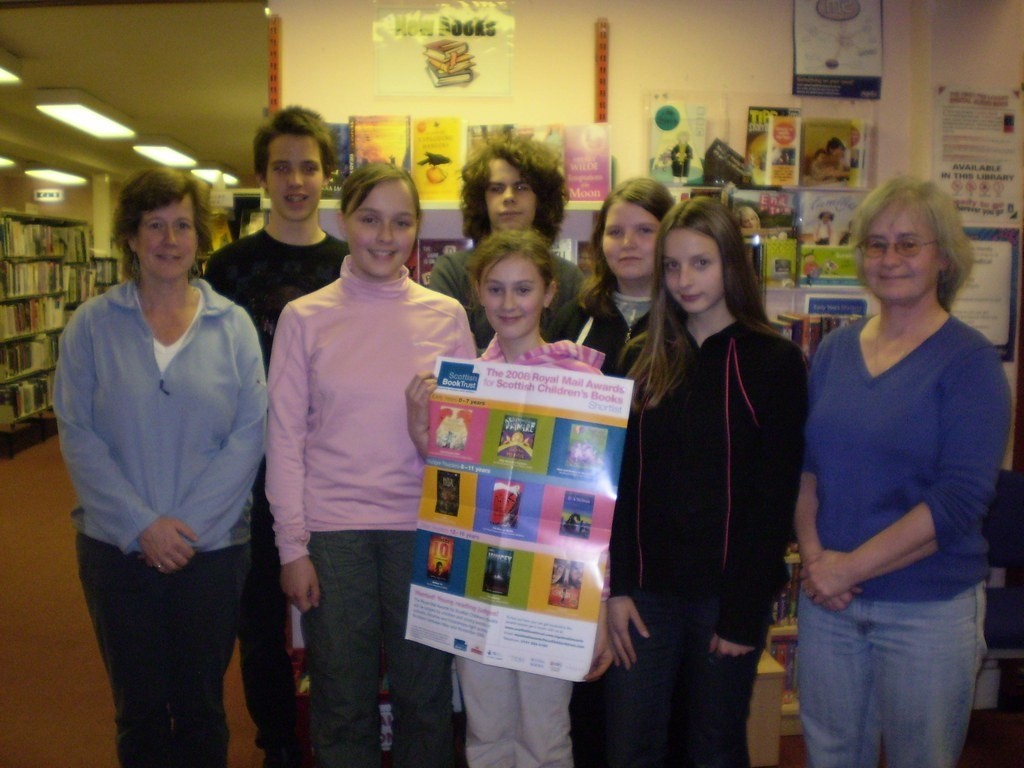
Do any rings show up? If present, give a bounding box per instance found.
[156,563,161,569]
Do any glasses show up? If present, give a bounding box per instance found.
[856,236,940,258]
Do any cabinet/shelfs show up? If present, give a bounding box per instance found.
[0,210,95,458]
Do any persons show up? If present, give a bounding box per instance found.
[792,179,1013,768]
[405,227,614,768]
[428,130,677,767]
[263,162,476,768]
[737,206,762,229]
[814,211,834,245]
[604,194,811,768]
[204,107,352,768]
[826,137,850,177]
[811,149,835,178]
[51,165,269,767]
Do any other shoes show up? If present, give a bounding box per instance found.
[255,728,304,768]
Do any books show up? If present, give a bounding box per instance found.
[0,215,114,424]
[771,553,800,704]
[690,188,873,288]
[650,102,708,186]
[467,123,612,202]
[319,115,412,200]
[769,292,870,354]
[800,118,872,187]
[411,115,463,202]
[744,105,803,186]
